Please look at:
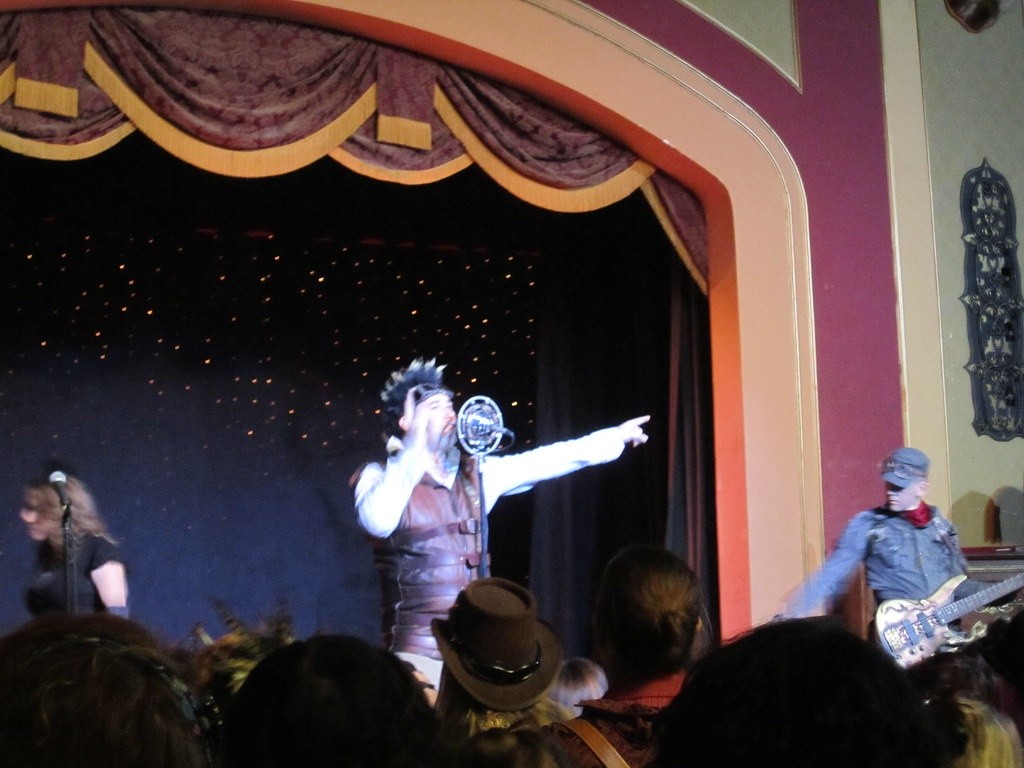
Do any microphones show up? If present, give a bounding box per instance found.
[457,396,514,455]
[49,472,71,508]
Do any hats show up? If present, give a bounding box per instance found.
[881,448,930,488]
[431,577,565,711]
[380,356,454,420]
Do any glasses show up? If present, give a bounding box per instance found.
[876,457,927,480]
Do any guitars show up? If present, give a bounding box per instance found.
[874,567,1024,668]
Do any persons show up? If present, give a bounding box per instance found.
[21,472,130,624]
[0,550,1024,768]
[773,448,1024,672]
[349,357,652,709]
